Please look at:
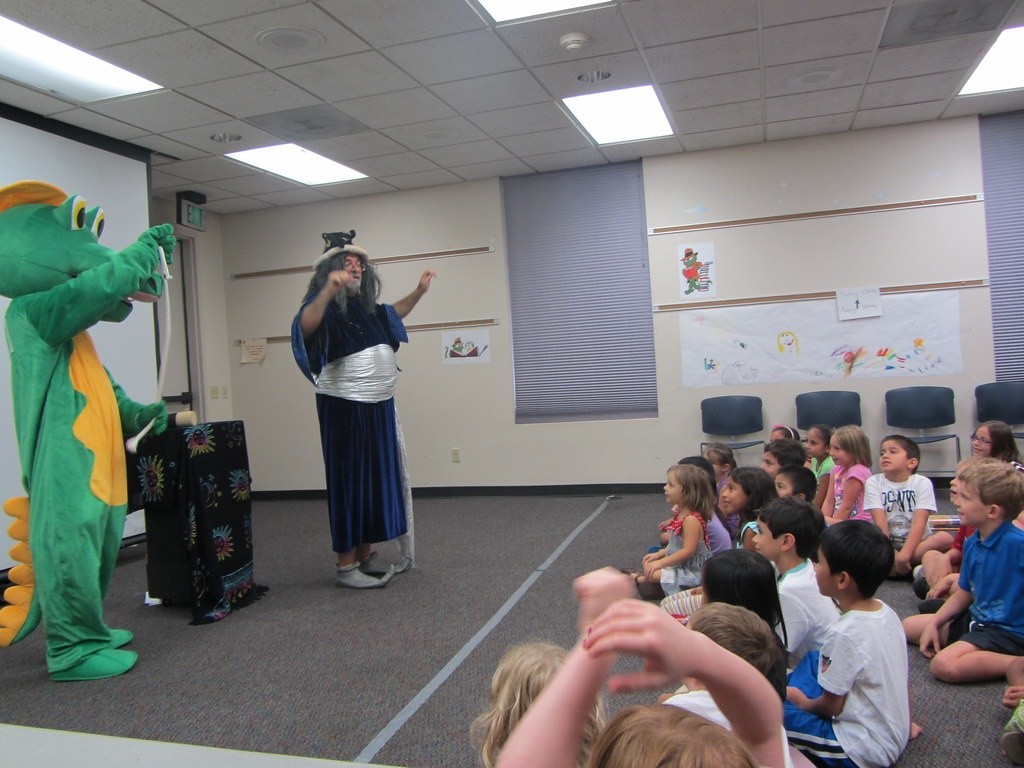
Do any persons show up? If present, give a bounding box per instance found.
[475,424,1024,768]
[290,230,437,587]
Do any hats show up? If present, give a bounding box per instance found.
[312,230,369,271]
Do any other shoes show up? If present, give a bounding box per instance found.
[647,546,661,553]
[359,550,411,575]
[913,565,925,579]
[621,568,644,584]
[334,561,395,588]
[918,599,946,614]
[1000,729,1024,766]
[639,582,665,600]
[913,576,930,600]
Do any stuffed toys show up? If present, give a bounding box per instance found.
[0,180,176,681]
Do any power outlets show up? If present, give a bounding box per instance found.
[452,448,461,462]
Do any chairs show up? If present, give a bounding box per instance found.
[796,390,863,434]
[974,382,1024,449]
[699,396,766,455]
[884,385,962,458]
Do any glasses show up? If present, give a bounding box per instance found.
[344,263,366,272]
[970,435,992,445]
